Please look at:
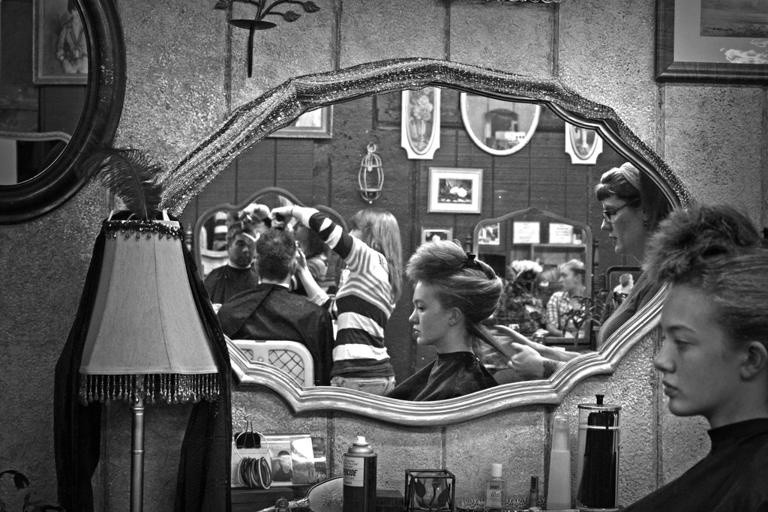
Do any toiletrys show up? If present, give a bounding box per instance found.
[526,472,542,509]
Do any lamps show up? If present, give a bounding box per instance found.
[77,219,220,512]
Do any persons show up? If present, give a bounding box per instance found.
[385,239,505,402]
[619,201,768,511]
[202,221,258,304]
[493,161,673,380]
[215,225,334,386]
[271,205,405,397]
[284,220,330,293]
[530,258,586,343]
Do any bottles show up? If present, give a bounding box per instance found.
[526,475,543,508]
[575,393,621,511]
[342,434,377,512]
[545,414,575,510]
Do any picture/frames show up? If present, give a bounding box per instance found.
[32,0,88,85]
[654,0,768,85]
[426,167,484,215]
[400,86,442,161]
[263,105,334,139]
[421,225,453,245]
[563,119,604,165]
[459,89,541,156]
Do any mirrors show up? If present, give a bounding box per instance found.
[192,186,352,316]
[605,265,646,299]
[472,206,594,346]
[0,0,128,225]
[154,59,704,430]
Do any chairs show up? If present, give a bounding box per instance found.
[232,339,314,388]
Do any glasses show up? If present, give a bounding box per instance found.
[602,198,641,220]
